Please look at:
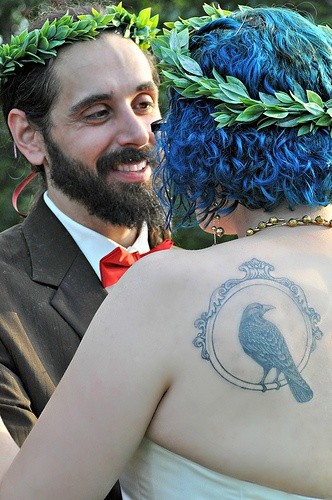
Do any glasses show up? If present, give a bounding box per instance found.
[151,118,170,148]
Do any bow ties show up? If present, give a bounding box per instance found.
[99,238,174,289]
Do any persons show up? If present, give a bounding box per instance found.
[0,1,172,500]
[1,0,332,499]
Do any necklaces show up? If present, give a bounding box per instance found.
[241,214,332,239]
